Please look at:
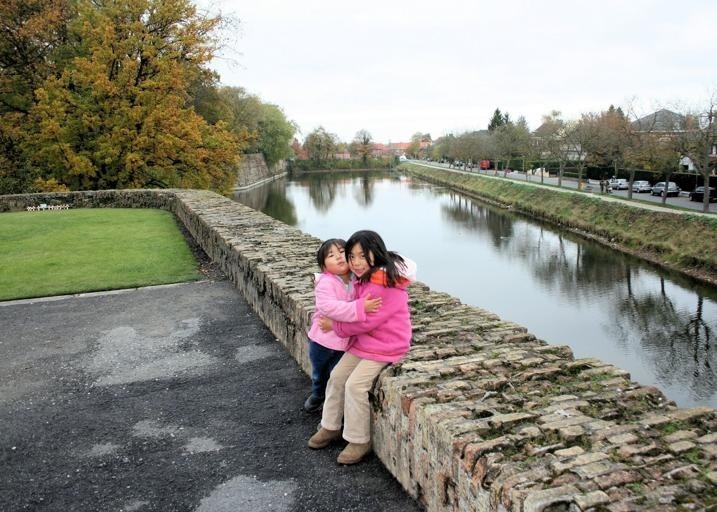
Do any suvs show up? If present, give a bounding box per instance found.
[651,182,679,196]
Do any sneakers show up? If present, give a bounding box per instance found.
[336,440,372,465]
[307,427,341,450]
[304,394,325,412]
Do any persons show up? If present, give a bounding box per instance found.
[599,180,611,192]
[307,230,411,465]
[304,238,382,414]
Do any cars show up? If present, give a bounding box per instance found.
[611,178,629,190]
[455,161,474,168]
[689,186,717,203]
[631,181,651,192]
